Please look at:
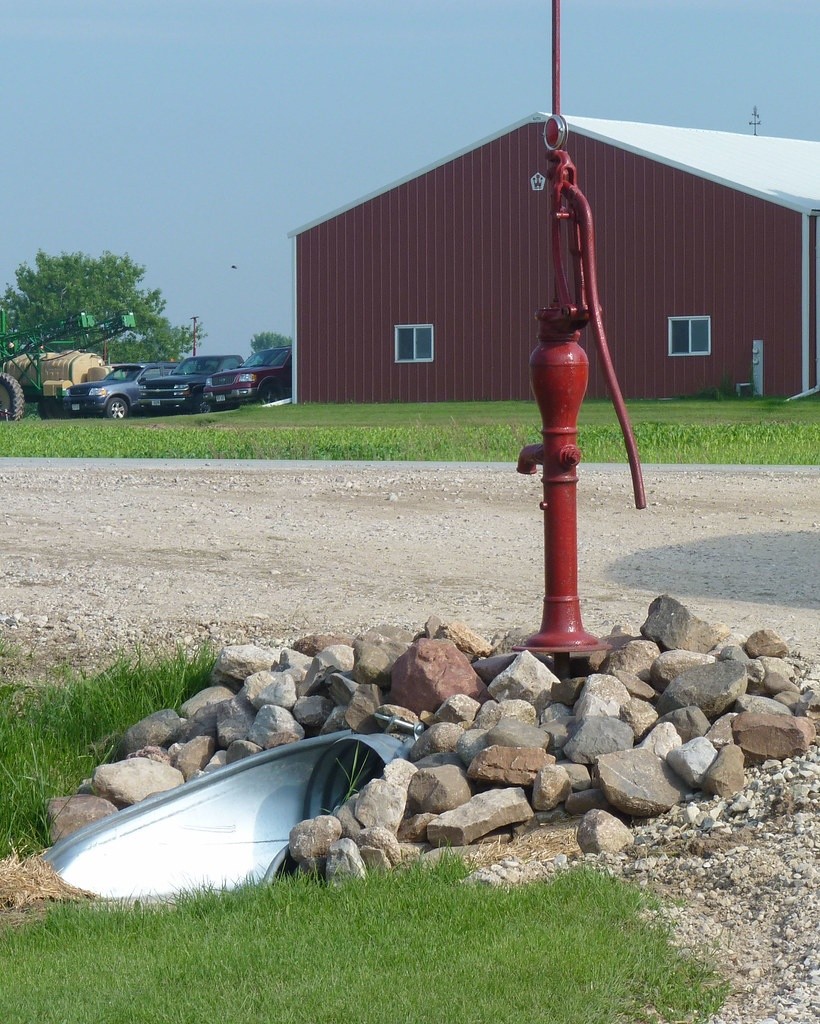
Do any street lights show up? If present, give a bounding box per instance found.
[191,316,199,356]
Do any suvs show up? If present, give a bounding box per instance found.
[63,363,179,419]
[137,354,245,414]
[202,344,292,411]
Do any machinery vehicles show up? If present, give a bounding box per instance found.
[0,304,136,421]
